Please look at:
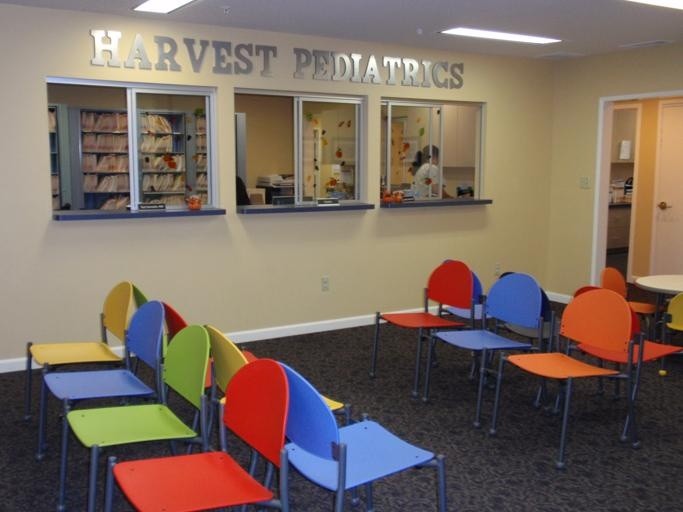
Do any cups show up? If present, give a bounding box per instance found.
[383,176,391,203]
[404,190,414,201]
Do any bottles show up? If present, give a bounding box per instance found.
[392,191,404,203]
[187,197,200,209]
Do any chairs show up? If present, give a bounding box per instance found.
[369,258,683,469]
[25,282,446,512]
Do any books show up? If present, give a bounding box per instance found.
[48,111,130,210]
[141,115,185,210]
[194,116,208,205]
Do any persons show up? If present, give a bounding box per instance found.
[413,145,454,200]
[236,175,252,206]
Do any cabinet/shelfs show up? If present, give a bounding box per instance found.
[46,101,71,212]
[66,103,191,210]
[187,108,209,202]
[257,185,294,204]
